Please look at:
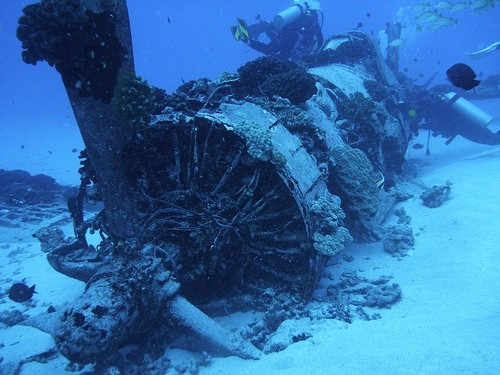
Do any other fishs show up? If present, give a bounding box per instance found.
[446,61,482,95]
[387,0,490,50]
[6,281,39,304]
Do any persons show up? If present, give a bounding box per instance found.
[230,9,324,57]
